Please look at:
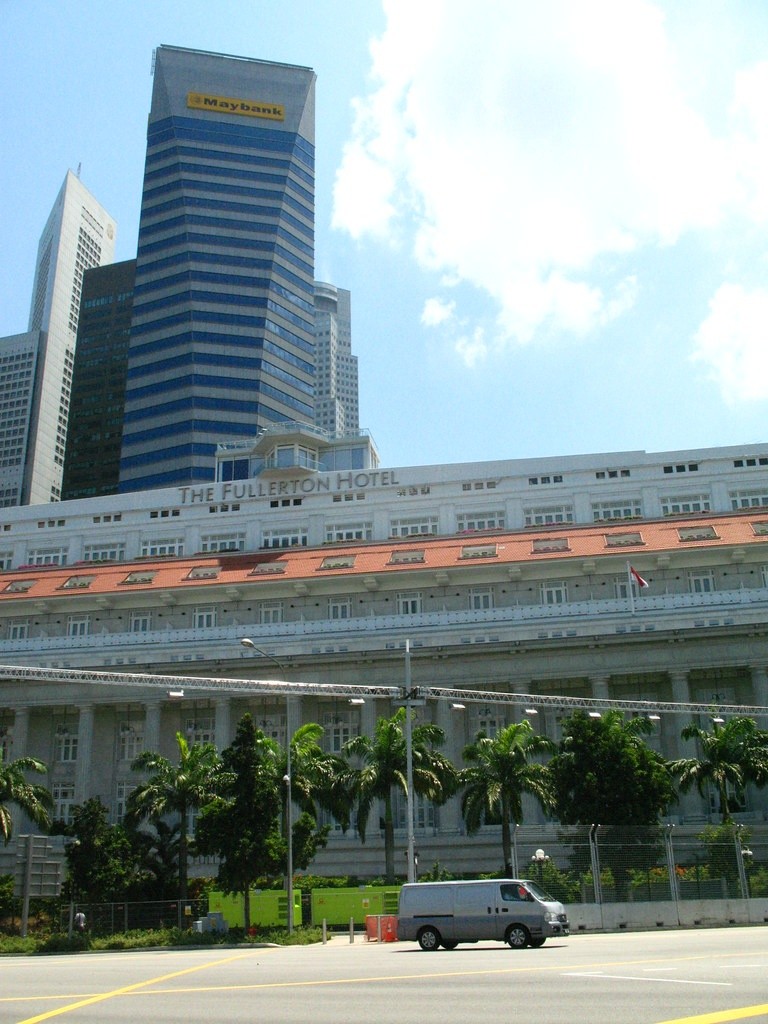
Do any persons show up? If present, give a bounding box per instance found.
[75,910,85,932]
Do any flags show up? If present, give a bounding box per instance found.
[630,567,649,588]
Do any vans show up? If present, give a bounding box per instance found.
[399,879,571,952]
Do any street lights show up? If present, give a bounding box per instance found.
[240,637,295,933]
[741,849,753,899]
[532,849,550,889]
